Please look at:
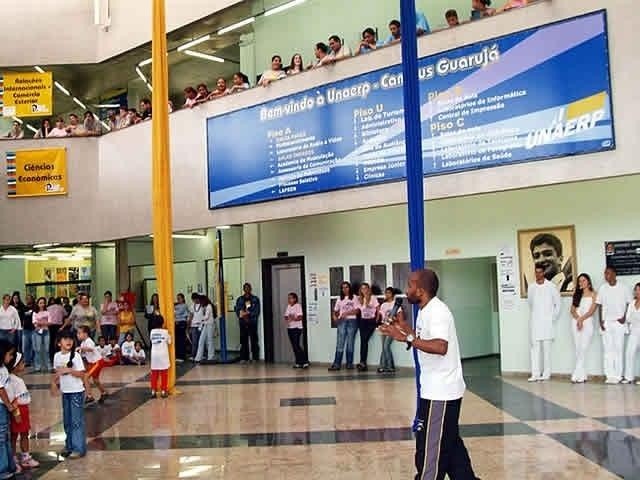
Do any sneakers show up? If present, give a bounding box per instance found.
[84,392,109,404]
[2,455,40,480]
[149,391,167,397]
[327,362,394,373]
[61,446,82,459]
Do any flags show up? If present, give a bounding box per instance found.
[5,146,69,197]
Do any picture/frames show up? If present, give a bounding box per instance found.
[514,221,581,300]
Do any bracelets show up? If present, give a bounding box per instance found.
[352,311,354,315]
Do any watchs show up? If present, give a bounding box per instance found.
[405,334,415,351]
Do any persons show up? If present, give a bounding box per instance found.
[283,292,310,370]
[74,326,107,403]
[168,72,250,113]
[569,272,598,383]
[1,290,160,373]
[594,267,631,384]
[173,292,218,366]
[622,282,640,384]
[233,283,262,362]
[530,234,573,292]
[471,1,528,17]
[327,281,361,372]
[355,10,432,56]
[376,287,404,373]
[376,269,482,479]
[1,338,40,479]
[527,265,561,382]
[149,314,172,399]
[6,99,152,138]
[446,9,460,28]
[258,35,352,86]
[357,283,379,372]
[49,329,88,458]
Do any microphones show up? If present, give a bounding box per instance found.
[386,296,403,326]
